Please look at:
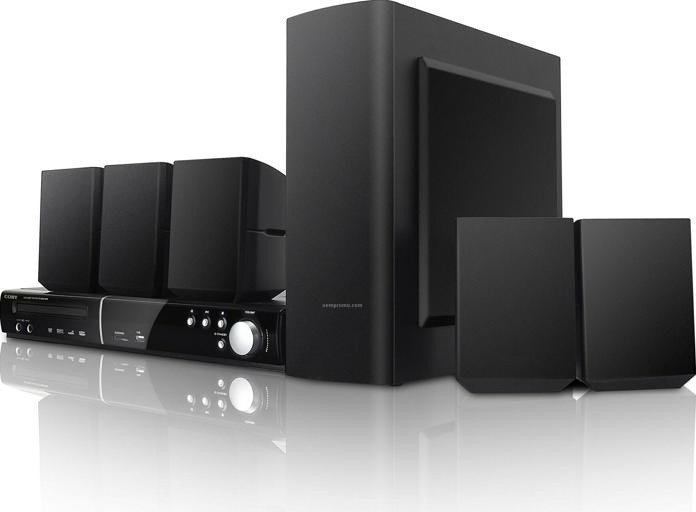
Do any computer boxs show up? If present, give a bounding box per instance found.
[286,0,564,392]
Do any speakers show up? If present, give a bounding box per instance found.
[100,163,166,297]
[582,219,695,394]
[38,166,102,295]
[459,217,576,395]
[168,157,288,301]
